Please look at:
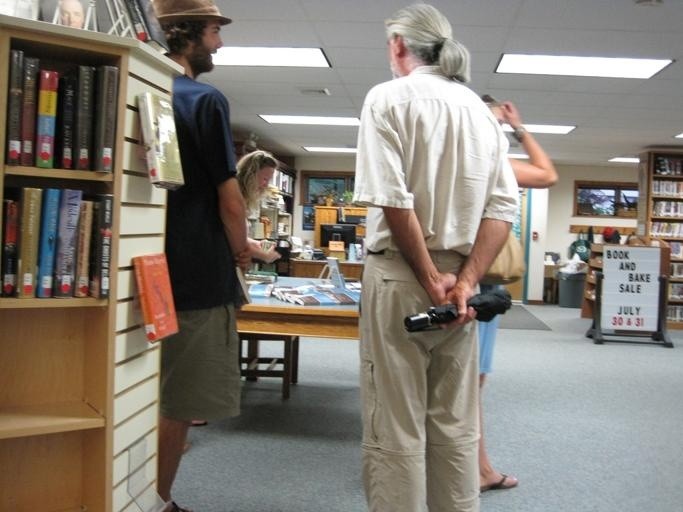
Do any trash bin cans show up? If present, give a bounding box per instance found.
[555,269,586,308]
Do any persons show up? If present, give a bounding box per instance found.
[150,1,255,510]
[182,149,283,453]
[351,4,519,512]
[476,94,560,492]
[60,1,84,28]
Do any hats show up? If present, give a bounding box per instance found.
[151,0,232,26]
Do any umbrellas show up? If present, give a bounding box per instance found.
[404,287,513,333]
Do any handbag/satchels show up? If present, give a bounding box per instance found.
[571,231,590,261]
[480,231,526,285]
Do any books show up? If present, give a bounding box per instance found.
[0,187,114,300]
[5,49,118,173]
[245,257,361,307]
[140,92,185,189]
[235,264,253,310]
[652,155,683,322]
[133,253,179,343]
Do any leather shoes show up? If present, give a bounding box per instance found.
[192,419,207,425]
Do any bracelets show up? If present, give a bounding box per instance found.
[514,125,527,142]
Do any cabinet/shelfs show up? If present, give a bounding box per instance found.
[311,206,367,258]
[0,16,132,512]
[580,145,683,331]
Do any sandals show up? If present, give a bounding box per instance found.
[480,474,519,492]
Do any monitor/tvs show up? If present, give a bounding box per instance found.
[320,225,355,246]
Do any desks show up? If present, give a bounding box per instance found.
[235,258,363,343]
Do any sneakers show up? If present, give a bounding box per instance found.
[163,501,193,512]
